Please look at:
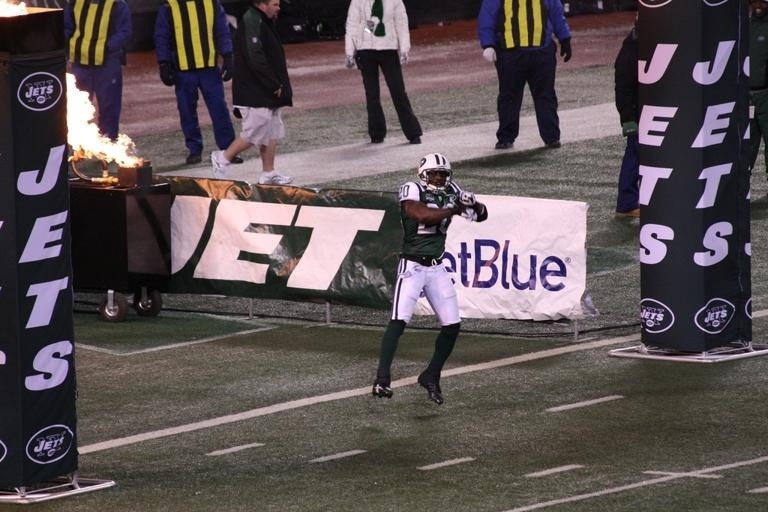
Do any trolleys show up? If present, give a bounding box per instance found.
[68,154,171,323]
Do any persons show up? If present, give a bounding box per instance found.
[210,0,294,184]
[344,0,422,144]
[154,0,243,164]
[372,153,487,404]
[612,10,642,218]
[477,0,572,149]
[62,0,134,143]
[743,1,767,181]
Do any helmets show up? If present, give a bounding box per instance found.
[417,152,453,194]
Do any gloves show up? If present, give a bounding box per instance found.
[560,36,571,62]
[446,203,478,222]
[159,63,178,87]
[220,51,234,81]
[457,190,479,208]
[623,120,638,137]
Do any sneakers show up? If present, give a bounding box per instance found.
[258,169,295,185]
[495,139,515,148]
[616,207,641,220]
[546,138,561,147]
[372,384,393,398]
[231,155,243,163]
[186,155,201,164]
[211,150,230,180]
[418,371,443,405]
